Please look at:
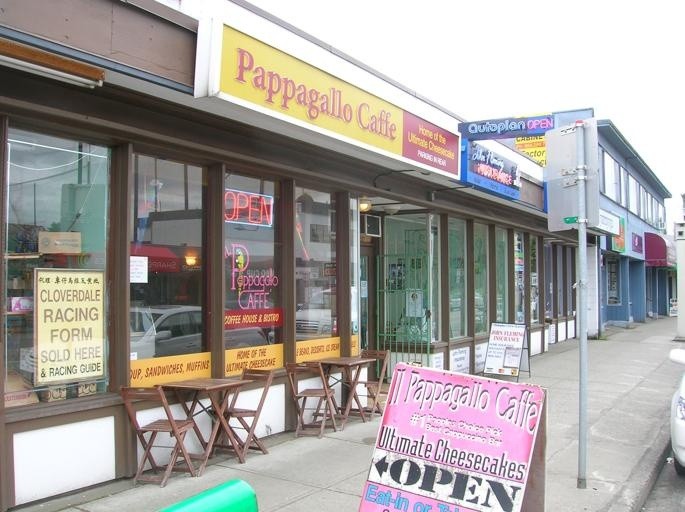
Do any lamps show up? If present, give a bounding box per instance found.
[358,199,374,211]
[383,205,401,215]
[371,168,433,192]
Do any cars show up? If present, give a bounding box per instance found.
[666,346,684,476]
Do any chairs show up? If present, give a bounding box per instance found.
[118,385,195,490]
[342,347,390,417]
[285,359,339,439]
[205,366,276,461]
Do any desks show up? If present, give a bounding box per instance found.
[307,354,378,431]
[159,372,253,476]
